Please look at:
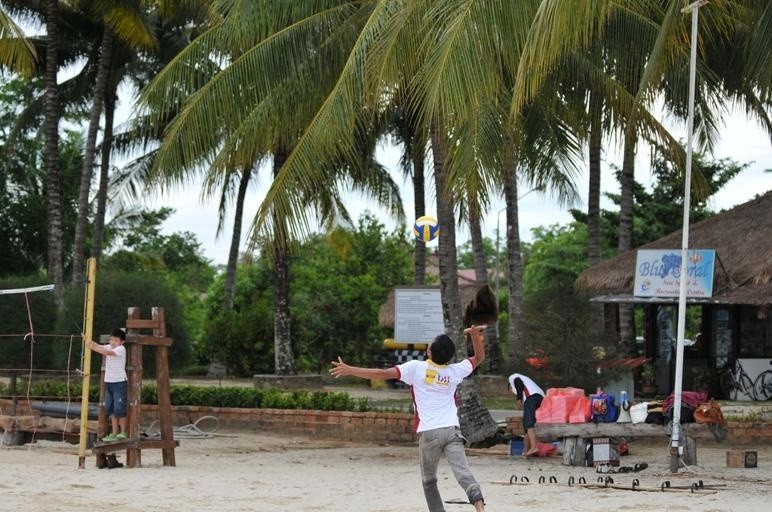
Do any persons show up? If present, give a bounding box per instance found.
[89,329,127,442]
[329,323,486,512]
[507,373,544,460]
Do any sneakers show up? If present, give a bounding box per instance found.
[100,432,129,443]
[96,453,123,470]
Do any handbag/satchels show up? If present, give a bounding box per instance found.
[693,398,725,426]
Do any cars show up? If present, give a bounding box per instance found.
[635,334,694,354]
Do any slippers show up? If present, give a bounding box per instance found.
[613,466,634,474]
[632,462,648,473]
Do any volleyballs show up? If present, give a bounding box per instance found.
[413,216,440,241]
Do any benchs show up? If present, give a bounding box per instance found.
[527,419,729,475]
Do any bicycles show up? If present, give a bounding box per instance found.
[716,348,772,404]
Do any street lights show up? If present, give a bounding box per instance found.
[493,179,555,345]
[666,0,715,479]
